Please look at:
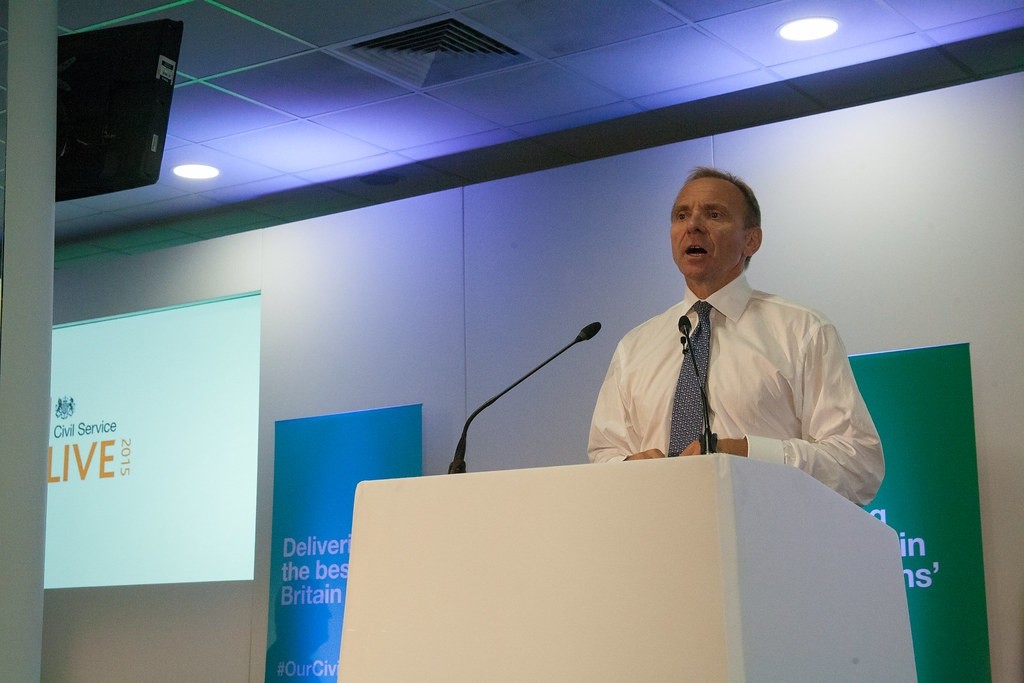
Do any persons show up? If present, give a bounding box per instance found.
[588,167,886,505]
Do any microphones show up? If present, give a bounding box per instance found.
[680,336,687,354]
[447,322,601,475]
[677,315,716,455]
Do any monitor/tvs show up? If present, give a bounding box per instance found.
[54,18,184,201]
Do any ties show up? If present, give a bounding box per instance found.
[668,301,713,458]
